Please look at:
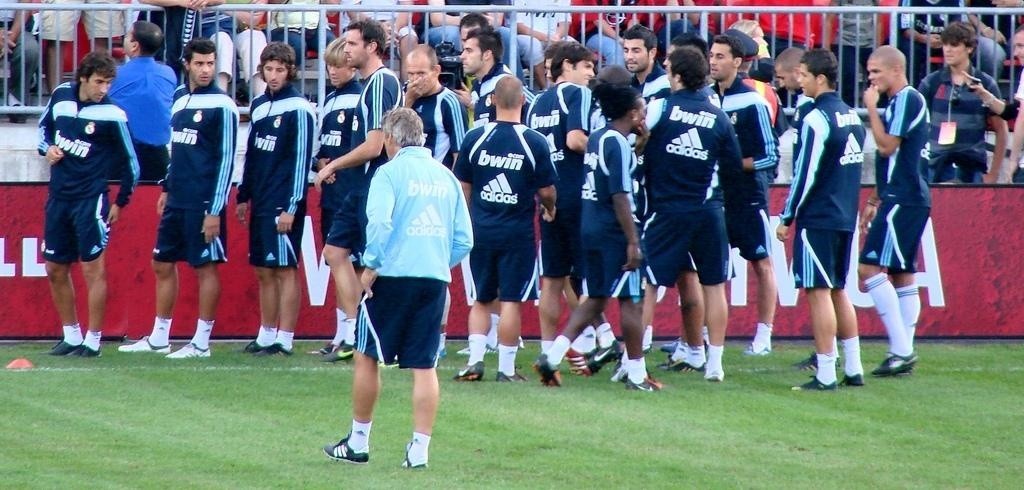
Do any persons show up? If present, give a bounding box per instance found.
[823,0,882,108]
[709,33,780,354]
[611,48,744,382]
[209,1,267,97]
[646,0,700,26]
[0,0,41,122]
[643,32,720,113]
[899,0,970,93]
[534,86,670,391]
[776,46,867,390]
[117,39,240,360]
[439,14,488,85]
[721,28,779,124]
[858,44,931,377]
[38,0,125,102]
[545,41,568,83]
[730,20,776,84]
[265,0,336,69]
[339,1,419,84]
[313,39,362,243]
[450,77,555,382]
[457,27,518,126]
[590,66,647,145]
[326,108,473,469]
[961,71,1018,123]
[919,21,1007,183]
[138,0,224,83]
[235,43,317,357]
[622,25,664,84]
[308,18,405,364]
[774,49,842,372]
[36,50,140,357]
[403,44,469,170]
[506,1,579,90]
[524,44,594,376]
[578,0,646,68]
[108,21,176,188]
[419,0,526,87]
[968,0,1016,46]
[1005,24,1024,185]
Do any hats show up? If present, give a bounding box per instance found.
[723,28,762,62]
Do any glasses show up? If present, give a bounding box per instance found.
[951,86,960,105]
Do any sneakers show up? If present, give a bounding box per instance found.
[588,339,624,374]
[742,343,773,356]
[564,347,592,377]
[254,343,293,357]
[39,337,86,356]
[401,442,429,468]
[703,362,725,382]
[666,360,708,374]
[656,353,676,368]
[791,351,842,371]
[165,343,212,359]
[322,433,369,465]
[870,348,919,376]
[496,366,529,383]
[790,375,839,392]
[452,360,485,381]
[531,354,562,387]
[456,344,497,355]
[118,335,172,354]
[838,372,866,387]
[625,376,665,393]
[611,346,630,382]
[661,335,708,354]
[64,343,102,358]
[319,340,355,362]
[233,340,269,354]
[304,340,339,356]
